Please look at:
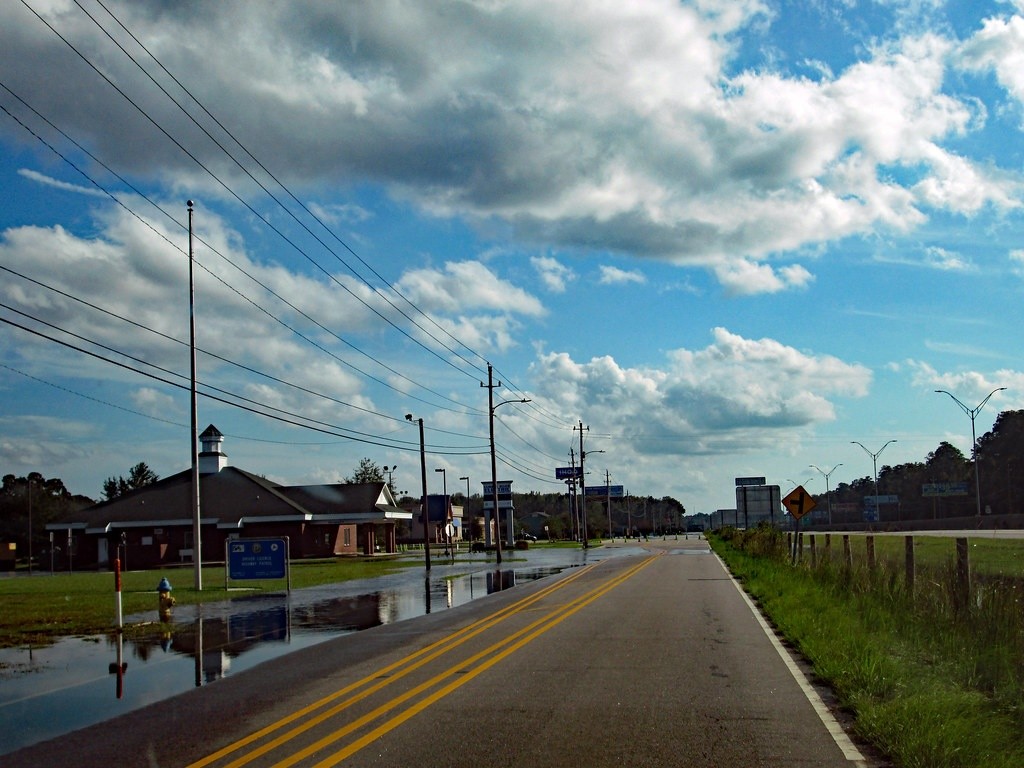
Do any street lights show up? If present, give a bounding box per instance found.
[489,398,532,564]
[786,478,813,525]
[808,464,845,527]
[385,465,398,496]
[849,440,899,523]
[935,388,1007,520]
[777,485,794,514]
[569,449,606,548]
[435,469,447,494]
[460,476,473,551]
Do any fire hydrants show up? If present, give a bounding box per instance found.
[155,575,177,622]
[160,628,174,655]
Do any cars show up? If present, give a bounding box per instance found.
[514,533,537,541]
